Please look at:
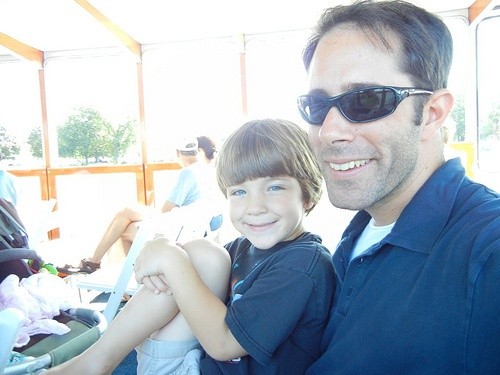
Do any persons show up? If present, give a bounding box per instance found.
[39,120,334,375]
[291,2,500,375]
[0,135,222,305]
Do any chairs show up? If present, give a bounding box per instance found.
[25,192,231,327]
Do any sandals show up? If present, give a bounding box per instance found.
[56,259,100,275]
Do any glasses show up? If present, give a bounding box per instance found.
[296,86,434,125]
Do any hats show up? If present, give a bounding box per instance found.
[176,135,198,151]
